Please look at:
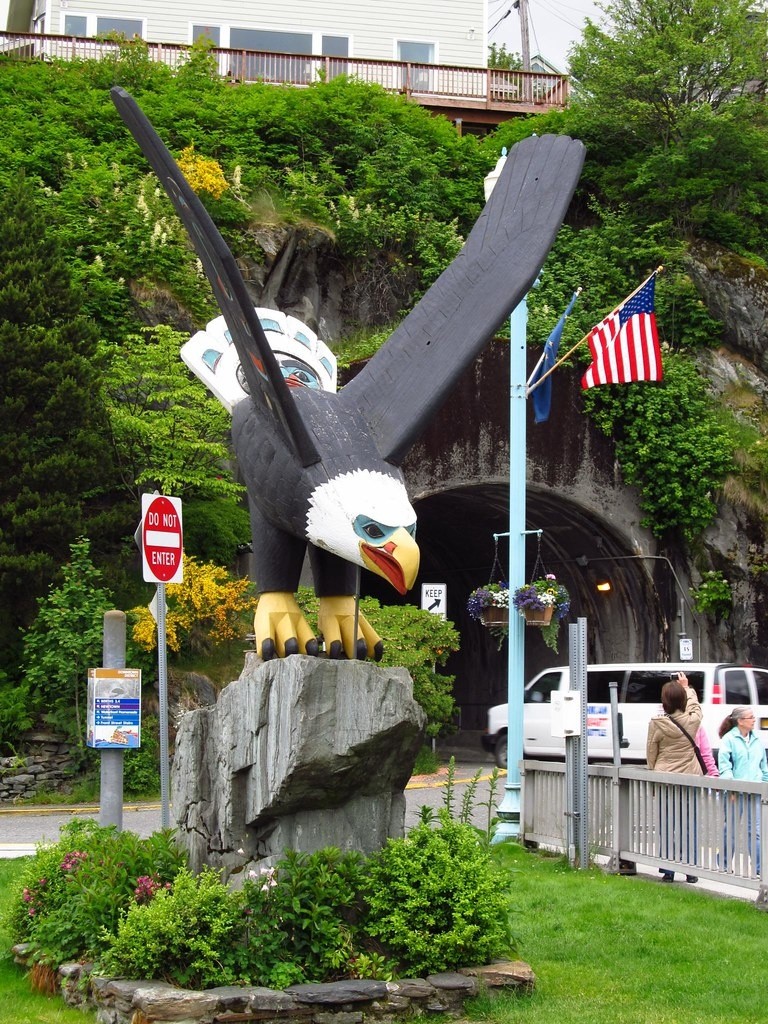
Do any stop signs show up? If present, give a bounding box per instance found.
[145,497,182,579]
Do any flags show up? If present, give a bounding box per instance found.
[581,272,663,391]
[531,291,577,427]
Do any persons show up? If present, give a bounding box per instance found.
[717,706,768,890]
[644,671,720,883]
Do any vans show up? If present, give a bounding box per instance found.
[482,662,767,776]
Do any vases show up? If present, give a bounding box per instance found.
[475,603,511,626]
[525,601,554,626]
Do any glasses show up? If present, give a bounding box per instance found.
[740,715,756,720]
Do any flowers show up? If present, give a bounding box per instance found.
[467,572,572,655]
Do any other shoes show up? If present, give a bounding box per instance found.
[662,873,673,883]
[687,874,697,883]
